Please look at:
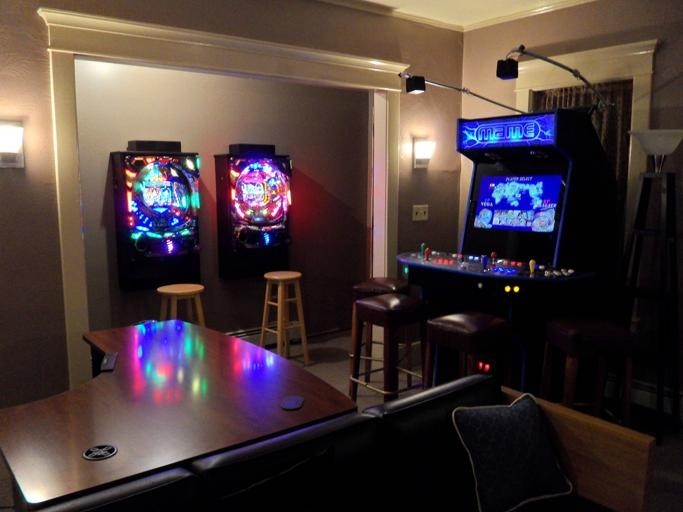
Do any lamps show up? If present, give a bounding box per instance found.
[615,129,683,446]
[398,70,527,114]
[496,43,613,113]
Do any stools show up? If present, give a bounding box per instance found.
[260,271,310,366]
[541,318,633,427]
[156,283,206,327]
[349,292,428,404]
[352,278,421,389]
[424,312,505,390]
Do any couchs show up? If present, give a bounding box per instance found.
[32,374,656,512]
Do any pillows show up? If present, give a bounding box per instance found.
[452,393,573,512]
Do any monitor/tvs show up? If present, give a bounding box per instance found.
[462,162,568,268]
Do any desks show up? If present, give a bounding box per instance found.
[0,320,357,512]
